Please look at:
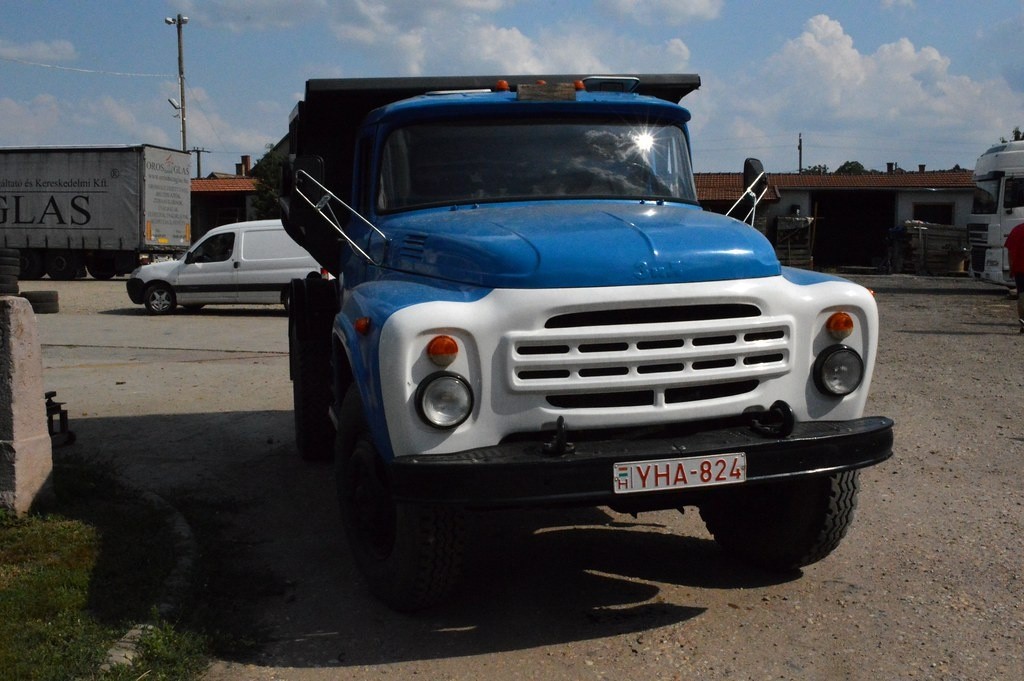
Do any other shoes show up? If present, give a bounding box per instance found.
[1018,317,1024,332]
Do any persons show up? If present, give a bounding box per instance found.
[1002,222,1024,335]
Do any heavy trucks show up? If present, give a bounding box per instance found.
[0,144,190,281]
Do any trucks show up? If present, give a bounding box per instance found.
[965,141,1024,290]
[254,73,892,620]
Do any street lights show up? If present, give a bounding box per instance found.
[164,14,190,151]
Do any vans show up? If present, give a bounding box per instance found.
[125,219,328,317]
